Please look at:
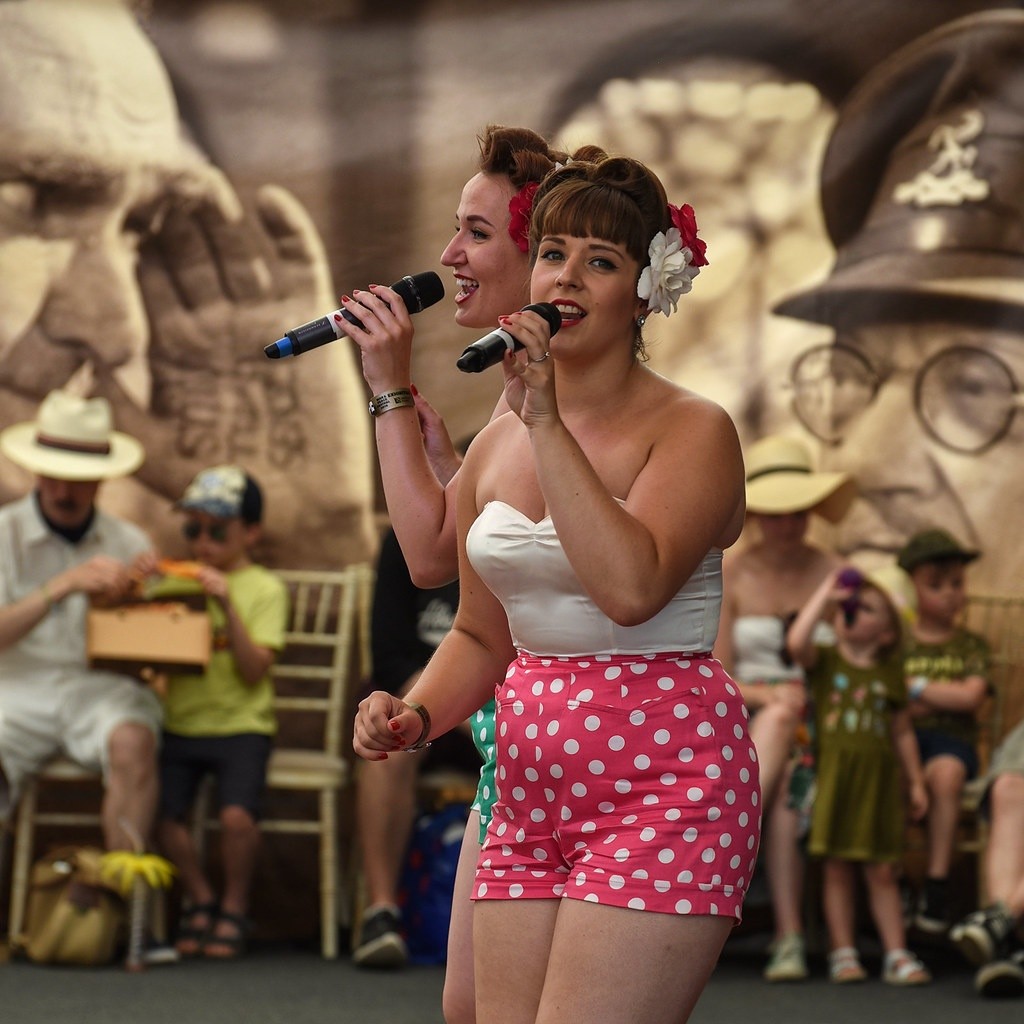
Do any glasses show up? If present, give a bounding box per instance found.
[774,610,799,670]
[179,517,243,541]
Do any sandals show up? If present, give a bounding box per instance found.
[205,910,251,965]
[179,899,218,963]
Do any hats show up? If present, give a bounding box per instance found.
[860,559,922,655]
[0,389,147,482]
[739,433,859,526]
[170,464,264,524]
[896,526,980,571]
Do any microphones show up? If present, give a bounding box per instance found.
[264,271,445,359]
[455,302,562,373]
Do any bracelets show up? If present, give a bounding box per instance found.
[43,583,54,610]
[401,701,432,753]
[369,387,416,417]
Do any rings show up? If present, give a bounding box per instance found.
[531,351,550,362]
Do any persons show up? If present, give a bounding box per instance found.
[897,529,999,937]
[945,717,1024,999]
[709,436,837,982]
[129,467,288,960]
[0,388,185,966]
[352,158,765,1023]
[785,548,932,987]
[332,127,571,1023]
[352,519,487,968]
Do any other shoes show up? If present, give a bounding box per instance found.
[765,871,1024,1001]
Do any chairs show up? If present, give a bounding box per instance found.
[3,750,165,960]
[176,561,367,963]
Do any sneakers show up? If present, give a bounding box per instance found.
[354,903,408,970]
[145,925,180,968]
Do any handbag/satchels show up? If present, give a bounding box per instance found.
[395,802,473,968]
[26,840,126,968]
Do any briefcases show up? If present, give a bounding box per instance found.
[84,562,214,675]
